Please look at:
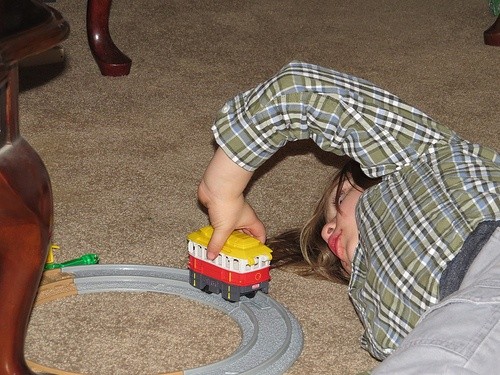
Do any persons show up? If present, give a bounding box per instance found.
[196,59,500,373]
